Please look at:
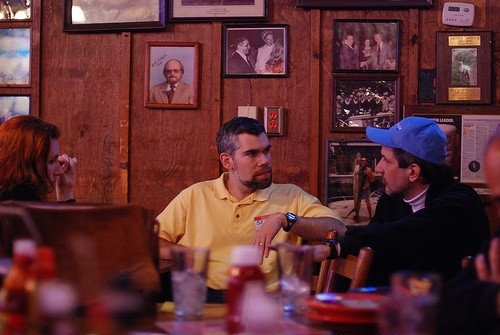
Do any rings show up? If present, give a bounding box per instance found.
[259,243,264,246]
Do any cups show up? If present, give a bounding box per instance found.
[169,246,210,322]
[277,244,315,316]
[378,270,443,335]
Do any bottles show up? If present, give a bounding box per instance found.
[1,240,45,335]
[224,245,267,334]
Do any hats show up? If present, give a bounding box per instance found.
[366,116,448,165]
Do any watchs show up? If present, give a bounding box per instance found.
[284,212,297,232]
[322,240,337,260]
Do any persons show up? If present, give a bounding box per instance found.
[228,30,284,73]
[353,157,375,221]
[338,86,395,128]
[334,31,395,70]
[150,116,348,302]
[150,59,193,104]
[0,115,78,205]
[456,56,464,82]
[267,117,489,294]
[439,128,500,335]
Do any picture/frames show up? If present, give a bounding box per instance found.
[63,0,166,33]
[328,73,403,140]
[222,23,290,78]
[323,138,384,227]
[435,29,493,106]
[332,18,402,73]
[145,41,200,109]
[168,0,269,23]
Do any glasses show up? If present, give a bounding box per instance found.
[163,69,182,74]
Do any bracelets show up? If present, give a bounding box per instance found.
[58,199,76,203]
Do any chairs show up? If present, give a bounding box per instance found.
[307,230,377,296]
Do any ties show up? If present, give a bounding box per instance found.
[167,84,176,104]
[376,44,380,66]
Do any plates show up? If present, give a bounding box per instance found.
[304,293,387,324]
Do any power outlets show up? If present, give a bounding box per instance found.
[264,106,285,136]
